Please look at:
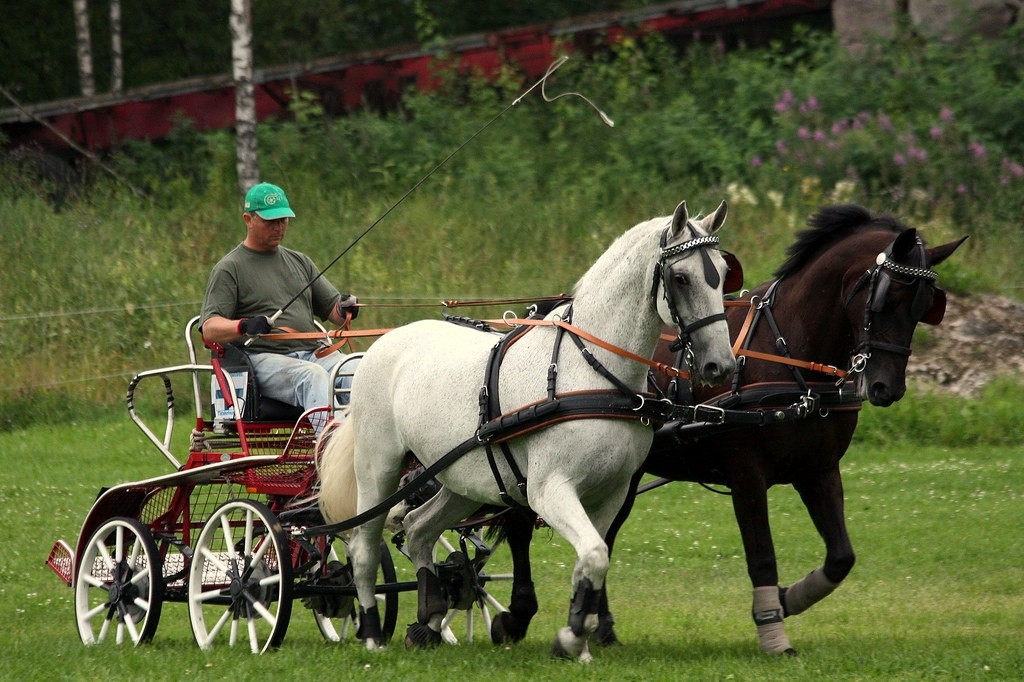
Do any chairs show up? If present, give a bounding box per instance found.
[154,316,337,431]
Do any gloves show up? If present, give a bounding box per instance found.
[237,315,275,335]
[338,294,359,320]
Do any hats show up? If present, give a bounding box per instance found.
[244,182,295,220]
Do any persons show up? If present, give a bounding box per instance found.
[201,182,368,440]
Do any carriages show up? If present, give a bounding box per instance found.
[45,199,967,660]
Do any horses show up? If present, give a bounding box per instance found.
[482,203,968,656]
[314,199,738,662]
